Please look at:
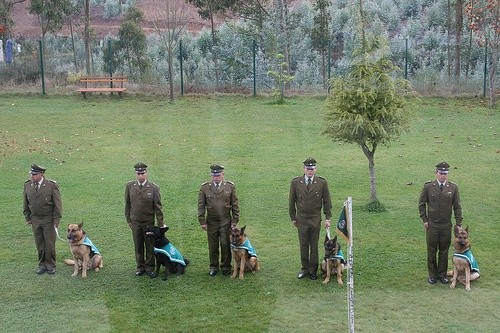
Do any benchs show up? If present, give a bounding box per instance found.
[76,76,127,99]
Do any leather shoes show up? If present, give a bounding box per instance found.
[310,274,317,280]
[147,272,153,276]
[36,269,45,274]
[222,270,231,275]
[47,270,56,274]
[428,278,437,283]
[135,271,142,276]
[440,277,448,284]
[209,270,217,276]
[298,272,305,279]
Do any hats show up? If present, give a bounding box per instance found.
[134,162,148,173]
[29,163,46,175]
[302,157,317,169]
[436,162,450,174]
[210,164,224,176]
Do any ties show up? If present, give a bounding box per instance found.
[35,183,39,194]
[139,183,143,190]
[306,179,311,191]
[216,184,219,191]
[439,183,444,192]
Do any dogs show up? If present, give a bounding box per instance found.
[144,225,190,281]
[320,234,347,285]
[63,221,103,277]
[229,225,260,280]
[446,225,480,291]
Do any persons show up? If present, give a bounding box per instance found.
[289,157,334,280]
[125,163,163,276]
[197,164,239,275]
[419,162,464,284]
[23,164,62,274]
